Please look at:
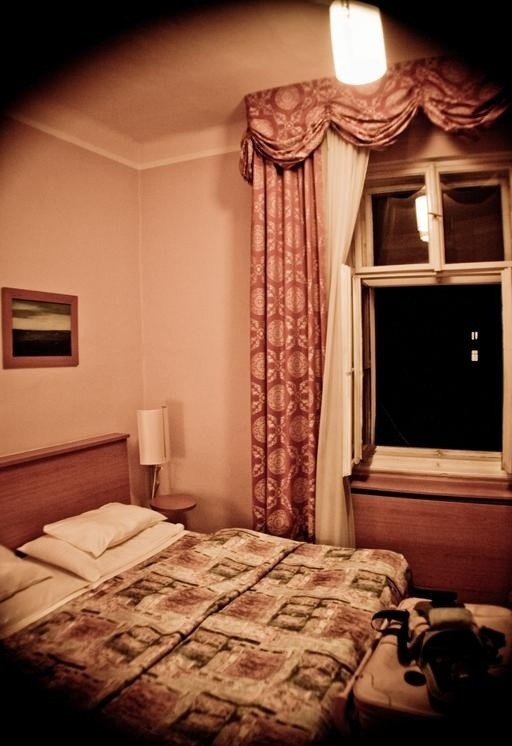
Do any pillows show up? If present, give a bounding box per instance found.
[0,502,185,637]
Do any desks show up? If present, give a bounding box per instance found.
[149,495,197,530]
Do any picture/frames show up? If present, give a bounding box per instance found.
[1,287,80,369]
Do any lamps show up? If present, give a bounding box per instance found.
[136,404,172,499]
[327,0,388,89]
[414,194,432,243]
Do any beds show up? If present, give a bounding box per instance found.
[1,431,412,746]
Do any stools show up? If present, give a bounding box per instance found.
[353,596,512,746]
